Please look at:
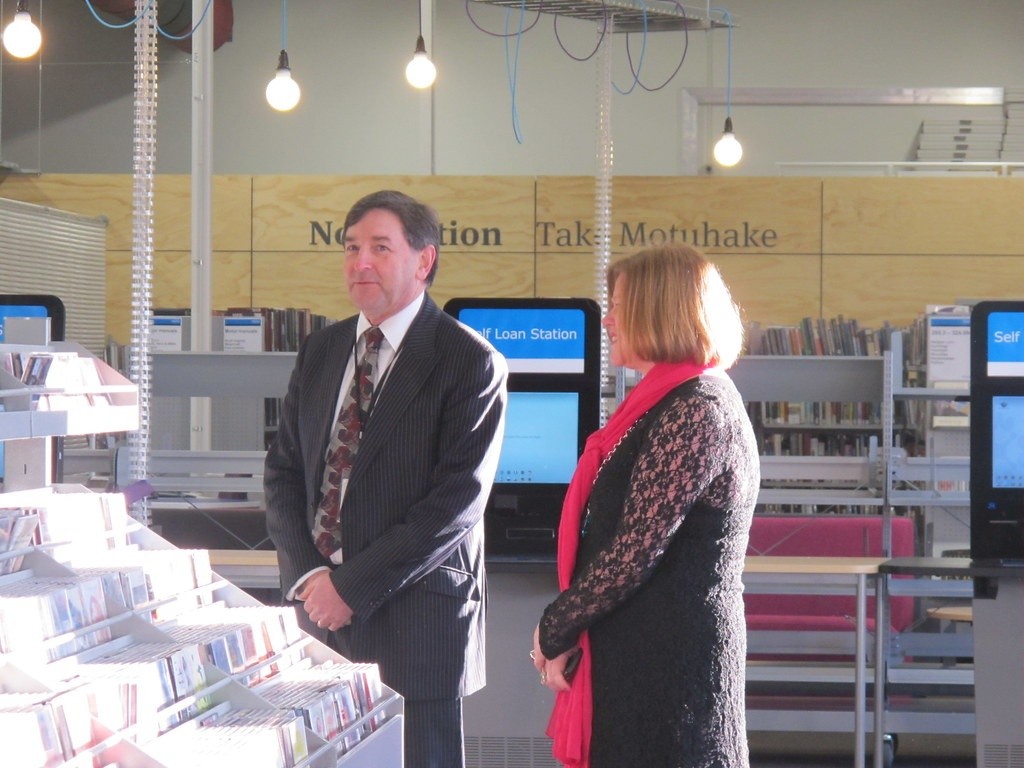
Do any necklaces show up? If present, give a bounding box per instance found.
[593,418,644,486]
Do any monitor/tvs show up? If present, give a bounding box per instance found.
[442,298,601,556]
[0,293,65,483]
[970,301,1024,562]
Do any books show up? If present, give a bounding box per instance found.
[740,314,927,514]
[0,352,113,409]
[0,488,387,768]
[212,307,339,425]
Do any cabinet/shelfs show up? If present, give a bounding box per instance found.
[724,330,1024,768]
[3,348,405,767]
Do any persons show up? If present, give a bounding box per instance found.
[262,189,507,767]
[534,245,761,768]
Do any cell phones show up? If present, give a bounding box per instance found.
[563,649,583,676]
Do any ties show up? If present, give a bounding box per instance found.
[311,326,385,558]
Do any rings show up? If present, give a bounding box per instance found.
[318,620,329,627]
[541,673,546,682]
[529,650,535,662]
